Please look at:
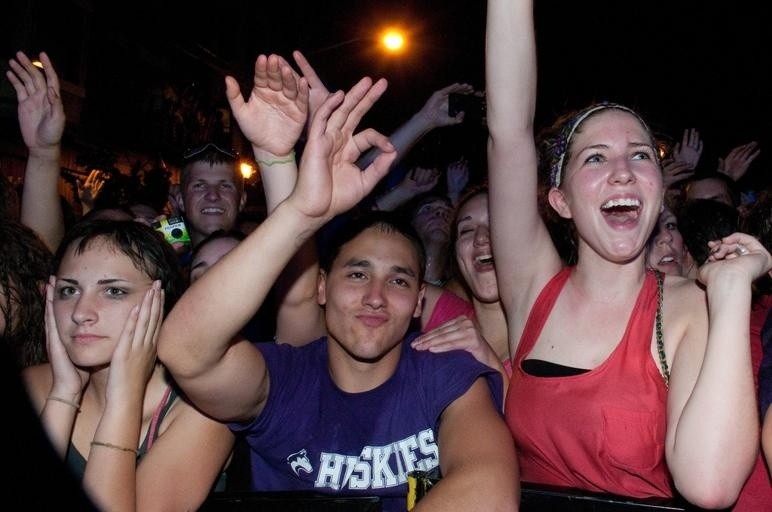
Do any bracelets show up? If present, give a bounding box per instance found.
[91,442,140,457]
[46,397,80,409]
[255,151,295,166]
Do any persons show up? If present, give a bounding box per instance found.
[487,0,772,512]
[21,217,243,511]
[644,127,771,510]
[157,77,520,512]
[0,50,515,410]
